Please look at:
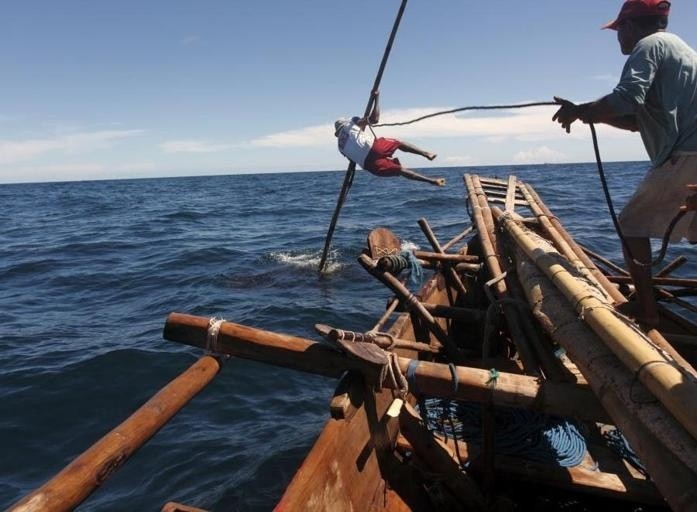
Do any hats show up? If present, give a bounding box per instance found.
[601,0,670,30]
[334,120,347,136]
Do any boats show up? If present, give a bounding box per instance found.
[268,216,694,512]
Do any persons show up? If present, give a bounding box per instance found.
[552,0,697,326]
[334,88,443,187]
